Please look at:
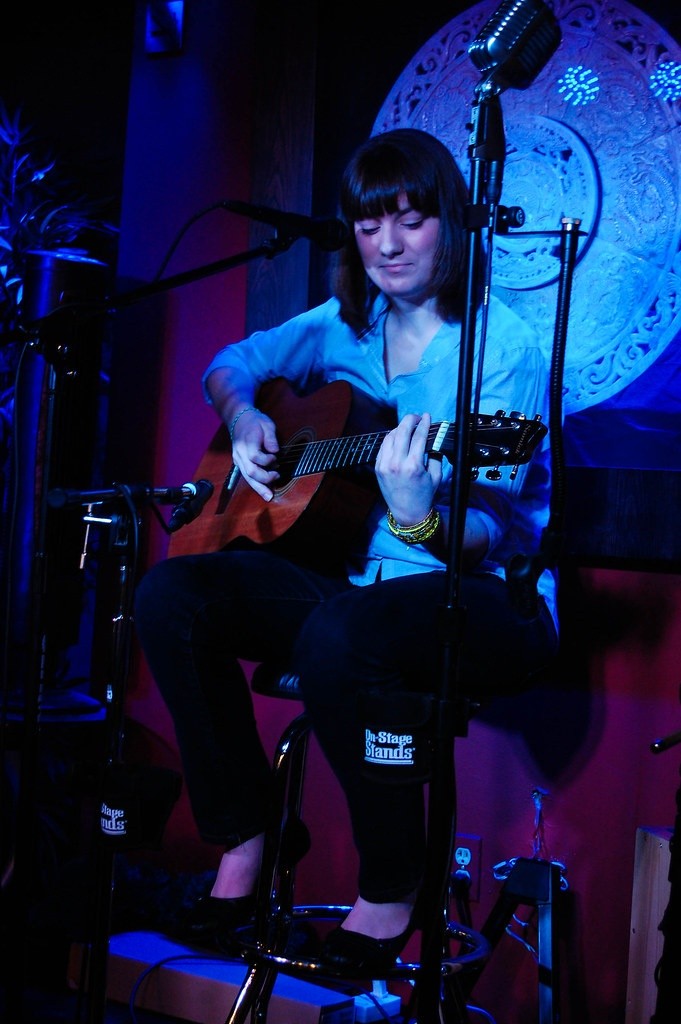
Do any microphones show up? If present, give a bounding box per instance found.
[471,0,563,204]
[220,198,349,254]
[168,479,215,532]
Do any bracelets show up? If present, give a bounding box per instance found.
[230,407,258,440]
[387,508,440,545]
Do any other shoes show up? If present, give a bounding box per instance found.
[184,829,278,933]
[322,892,422,971]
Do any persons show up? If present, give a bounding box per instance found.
[134,128,566,992]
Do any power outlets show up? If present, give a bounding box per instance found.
[448,833,482,903]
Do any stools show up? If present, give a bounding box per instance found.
[228,656,497,1024]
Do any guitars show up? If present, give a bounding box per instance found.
[166,375,549,564]
